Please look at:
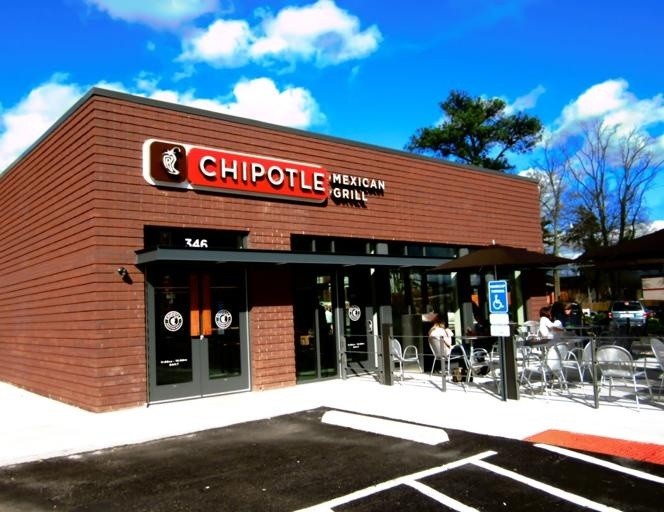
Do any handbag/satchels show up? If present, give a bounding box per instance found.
[453,368,473,382]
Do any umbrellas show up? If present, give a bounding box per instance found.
[426,239,576,281]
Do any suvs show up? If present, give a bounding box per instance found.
[552,300,583,326]
[608,300,651,325]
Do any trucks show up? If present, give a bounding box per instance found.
[581,307,599,321]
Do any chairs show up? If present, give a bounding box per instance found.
[376,325,663,411]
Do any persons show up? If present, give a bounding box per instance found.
[539,306,563,338]
[466,308,497,374]
[428,314,473,381]
[549,295,567,322]
